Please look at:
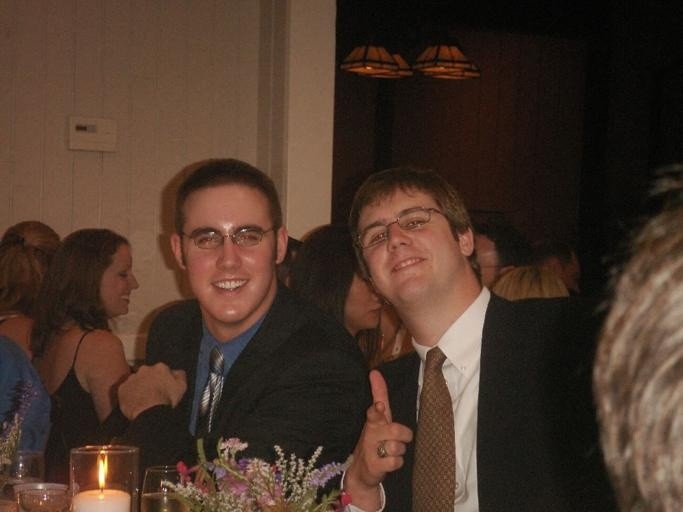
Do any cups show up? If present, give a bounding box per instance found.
[13,452,46,482]
[141,463,189,512]
[69,445,139,512]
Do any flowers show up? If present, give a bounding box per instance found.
[1,379,39,466]
[160,435,354,512]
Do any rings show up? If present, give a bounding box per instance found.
[377,443,386,458]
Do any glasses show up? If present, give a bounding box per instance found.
[351,205,453,250]
[179,224,275,250]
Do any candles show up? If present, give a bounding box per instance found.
[74,449,131,512]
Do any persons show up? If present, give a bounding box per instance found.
[279,214,586,369]
[117,162,372,503]
[29,227,139,484]
[592,162,683,510]
[336,163,619,511]
[0,219,61,361]
[1,334,53,472]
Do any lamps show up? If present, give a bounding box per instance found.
[340,30,480,80]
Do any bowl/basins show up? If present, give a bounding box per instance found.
[13,482,69,512]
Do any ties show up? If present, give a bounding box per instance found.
[194,347,224,475]
[411,347,456,510]
